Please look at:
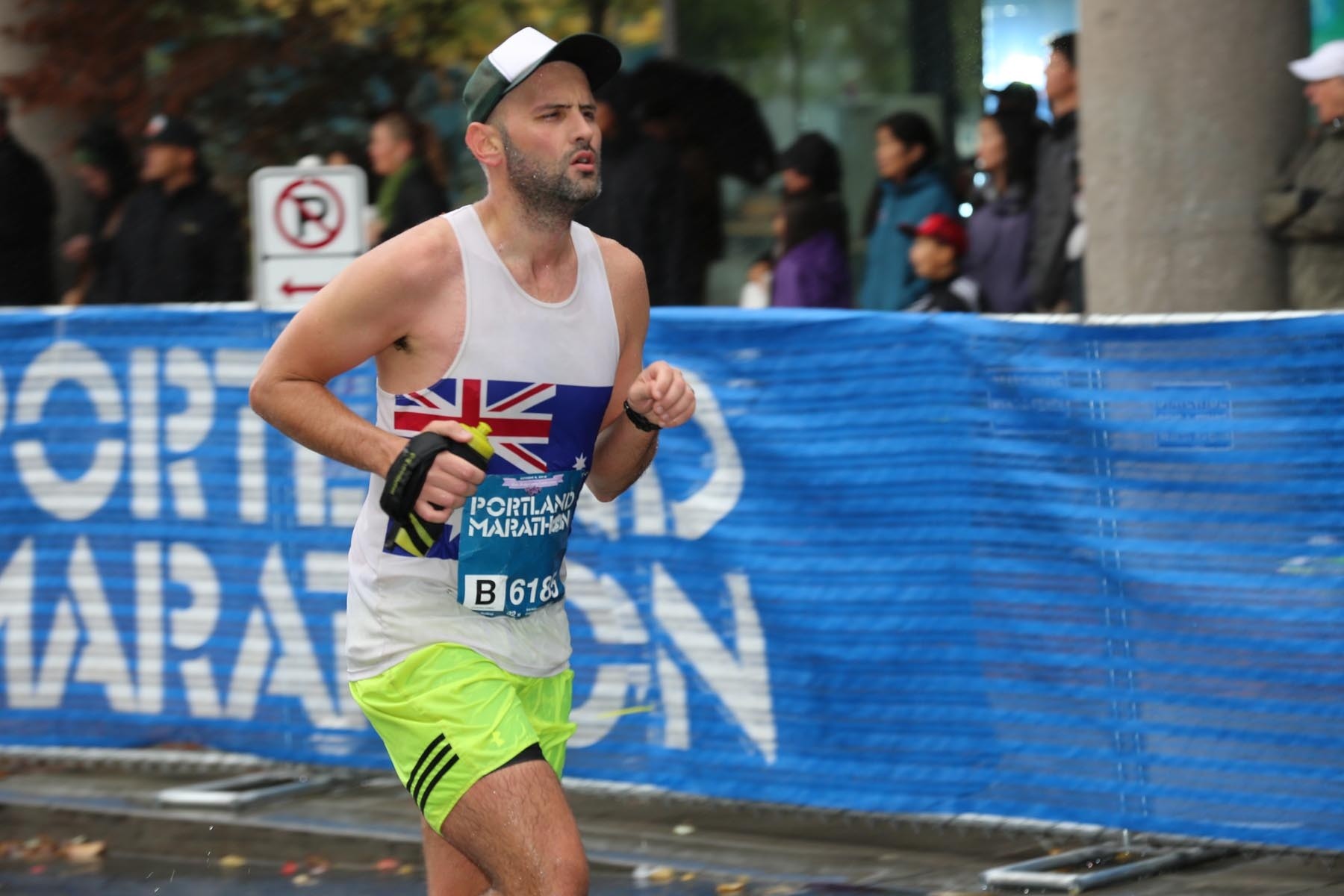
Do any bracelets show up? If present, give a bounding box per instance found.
[623,399,664,432]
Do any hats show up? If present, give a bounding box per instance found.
[460,27,622,125]
[1287,39,1344,82]
[986,82,1038,112]
[778,131,842,172]
[137,114,195,148]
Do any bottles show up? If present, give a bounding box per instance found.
[394,422,495,557]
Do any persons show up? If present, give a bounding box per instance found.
[1,0,1085,319]
[1257,39,1344,313]
[247,30,697,896]
